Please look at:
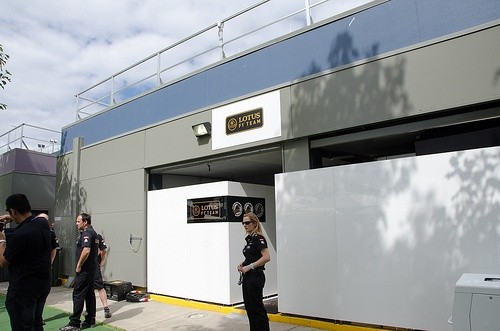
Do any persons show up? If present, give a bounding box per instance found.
[237,213,270,331]
[60,213,111,331]
[0,194,59,331]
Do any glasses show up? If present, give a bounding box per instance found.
[242,221,251,225]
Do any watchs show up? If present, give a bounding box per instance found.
[250,263,254,269]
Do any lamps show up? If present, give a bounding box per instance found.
[191,122,211,138]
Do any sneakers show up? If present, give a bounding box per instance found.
[83,310,95,316]
[60,324,80,331]
[104,306,111,318]
[80,320,96,327]
